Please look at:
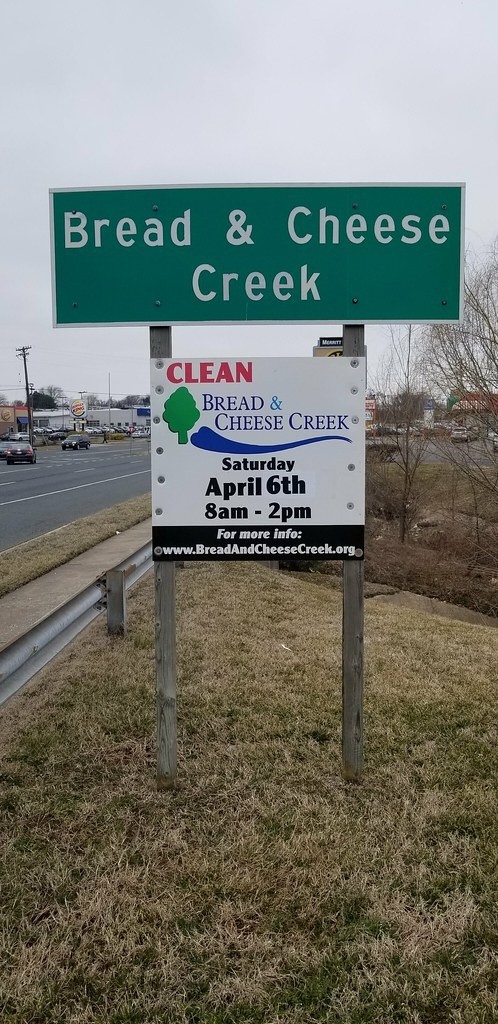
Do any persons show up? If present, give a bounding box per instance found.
[103,431,108,443]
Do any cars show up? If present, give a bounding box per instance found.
[48,432,67,441]
[365,420,467,439]
[493,435,498,452]
[10,432,36,442]
[0,432,18,441]
[6,443,36,465]
[0,441,19,459]
[32,425,151,434]
[131,430,149,439]
[61,434,90,450]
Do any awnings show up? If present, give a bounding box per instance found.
[17,417,29,424]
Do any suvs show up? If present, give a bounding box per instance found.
[451,427,480,444]
